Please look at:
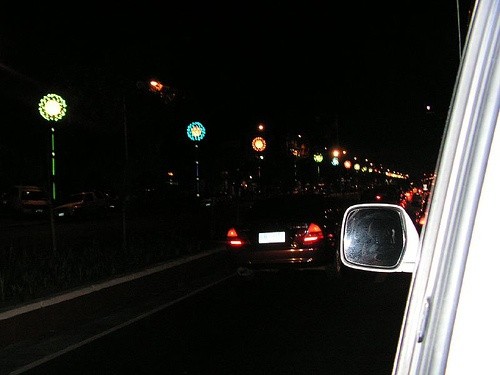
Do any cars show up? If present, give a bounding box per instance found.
[337,0,499,375]
[223,190,356,282]
[0,161,437,238]
